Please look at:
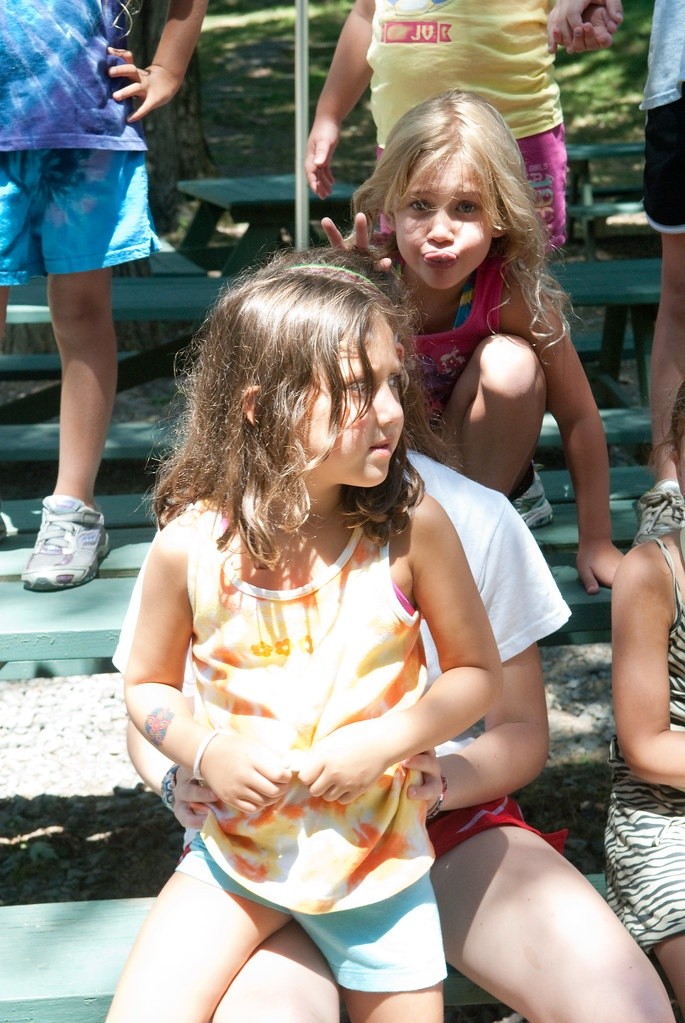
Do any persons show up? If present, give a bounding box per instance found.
[0,0,211,591]
[135,248,676,1023]
[604,381,685,1023]
[105,272,504,1023]
[322,89,625,594]
[304,0,685,548]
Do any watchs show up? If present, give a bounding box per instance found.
[161,764,181,812]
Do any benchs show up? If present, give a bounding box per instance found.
[0,172,662,1023]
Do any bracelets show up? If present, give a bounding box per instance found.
[426,775,448,820]
[193,731,220,781]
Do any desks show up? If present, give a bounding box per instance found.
[177,178,359,271]
[562,140,649,256]
[9,258,664,470]
[0,468,656,679]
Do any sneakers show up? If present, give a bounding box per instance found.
[510,460,554,528]
[632,479,685,548]
[21,495,110,590]
